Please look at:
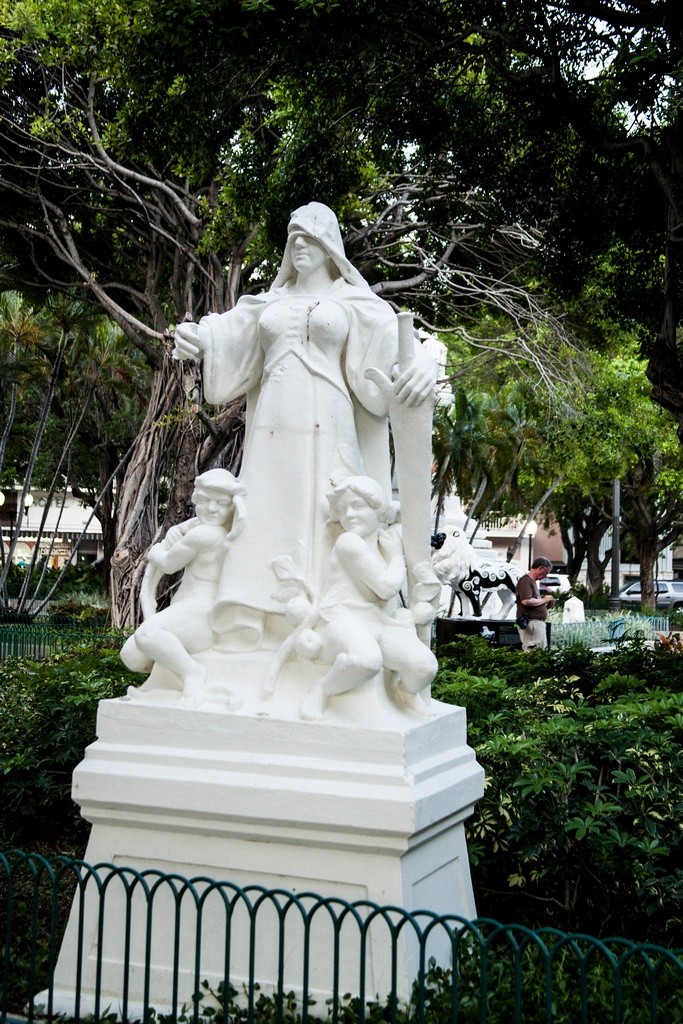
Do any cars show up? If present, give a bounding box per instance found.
[619,578,683,615]
[540,573,572,599]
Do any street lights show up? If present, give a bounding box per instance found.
[0,491,33,563]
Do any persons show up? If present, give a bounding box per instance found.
[116,469,243,702]
[308,470,439,722]
[37,554,47,571]
[179,199,438,667]
[516,556,556,654]
